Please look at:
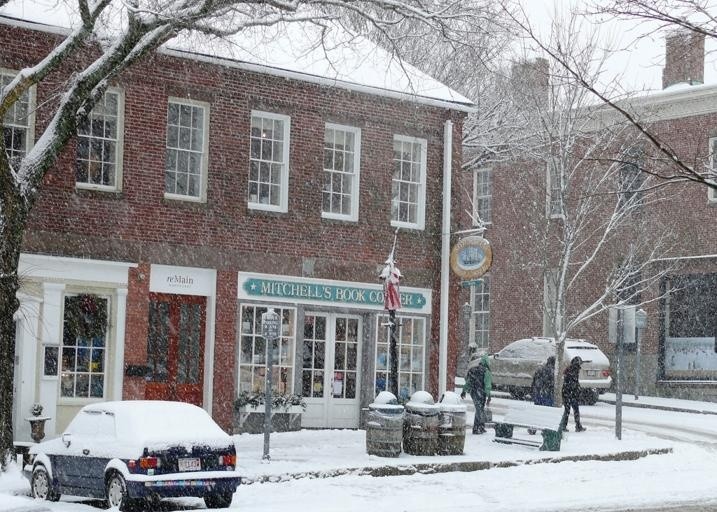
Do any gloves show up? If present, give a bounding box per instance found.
[461,392,466,399]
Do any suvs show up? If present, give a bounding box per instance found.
[466,337,612,405]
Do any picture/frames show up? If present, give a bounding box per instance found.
[39,343,61,381]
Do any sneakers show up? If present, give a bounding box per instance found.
[559,425,586,432]
[473,428,486,435]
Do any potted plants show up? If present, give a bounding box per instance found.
[232,390,307,430]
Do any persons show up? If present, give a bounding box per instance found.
[559,356,587,434]
[460,352,494,437]
[529,357,556,437]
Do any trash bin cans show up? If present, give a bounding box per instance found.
[366,391,465,456]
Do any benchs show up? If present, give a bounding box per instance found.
[486,402,569,451]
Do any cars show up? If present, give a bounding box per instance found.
[22,400,239,508]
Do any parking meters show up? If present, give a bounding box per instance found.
[25,403,52,446]
